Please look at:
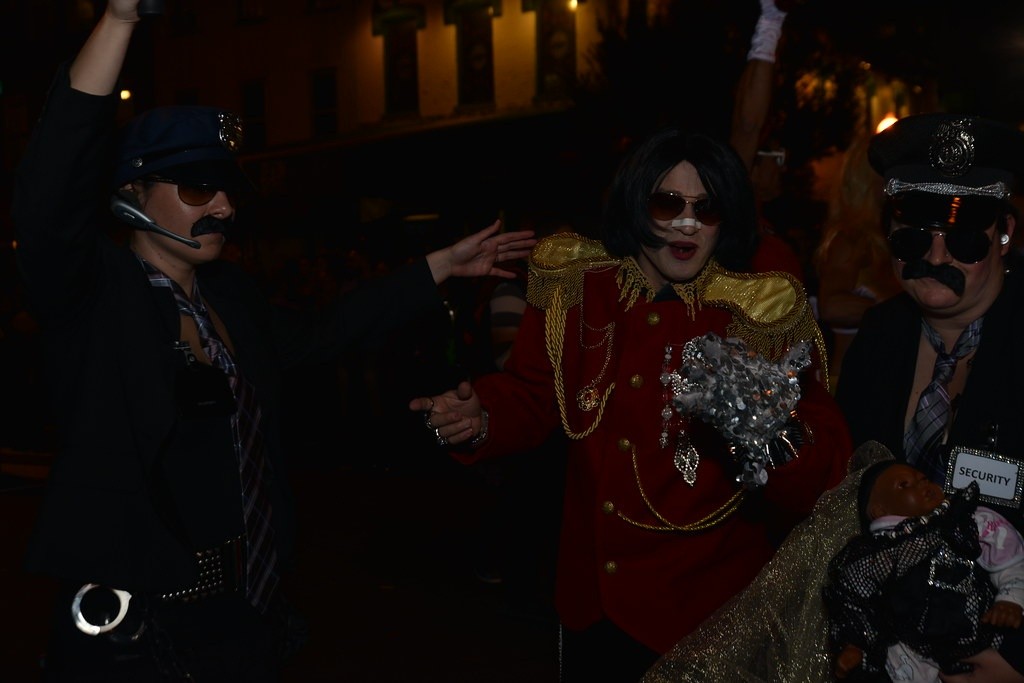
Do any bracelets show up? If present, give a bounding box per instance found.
[472,408,488,443]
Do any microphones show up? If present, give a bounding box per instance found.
[112,200,203,252]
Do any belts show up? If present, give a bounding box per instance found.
[148,533,248,602]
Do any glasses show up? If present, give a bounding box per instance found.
[647,193,726,227]
[882,214,1000,265]
[136,174,251,212]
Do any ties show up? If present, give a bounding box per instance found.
[141,255,279,616]
[903,314,985,467]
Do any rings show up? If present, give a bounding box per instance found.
[427,397,434,412]
[432,428,441,441]
[425,419,433,431]
[440,438,449,449]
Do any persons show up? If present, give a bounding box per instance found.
[835,113,1024,683]
[0,0,576,683]
[409,128,852,683]
[825,460,1024,683]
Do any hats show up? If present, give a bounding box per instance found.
[867,113,1024,235]
[111,105,245,190]
